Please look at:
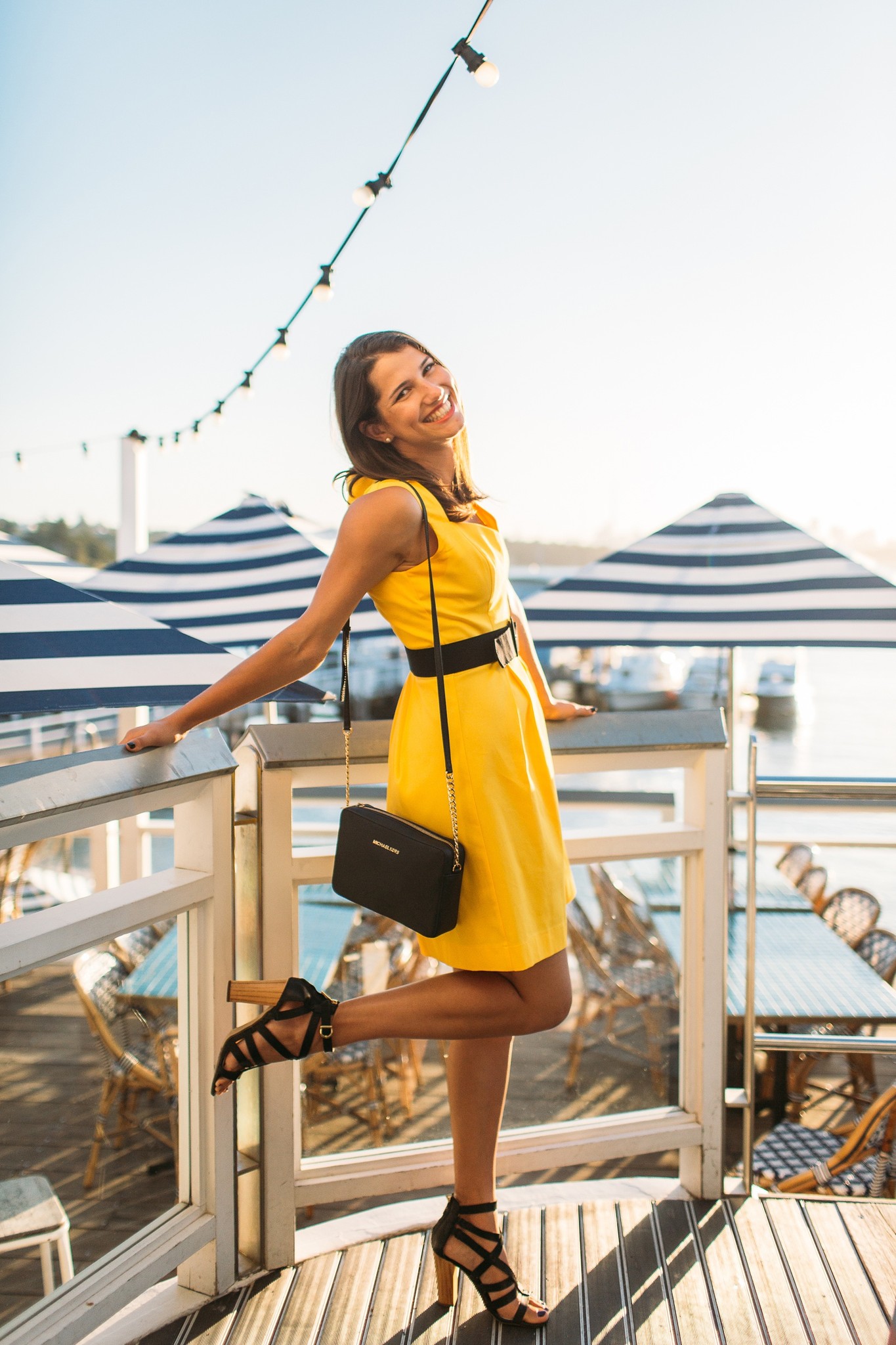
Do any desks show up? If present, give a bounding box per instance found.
[648,913,894,1124]
[625,859,815,915]
[115,904,361,1016]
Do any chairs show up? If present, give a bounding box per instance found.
[0,842,895,1303]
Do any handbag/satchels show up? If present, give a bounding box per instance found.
[331,803,465,939]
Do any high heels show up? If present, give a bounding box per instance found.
[430,1192,548,1328]
[210,977,341,1097]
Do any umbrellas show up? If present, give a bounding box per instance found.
[64,492,402,724]
[519,487,896,790]
[0,559,341,756]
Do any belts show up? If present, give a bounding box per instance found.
[406,617,520,678]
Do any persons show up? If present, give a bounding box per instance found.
[122,329,603,1327]
